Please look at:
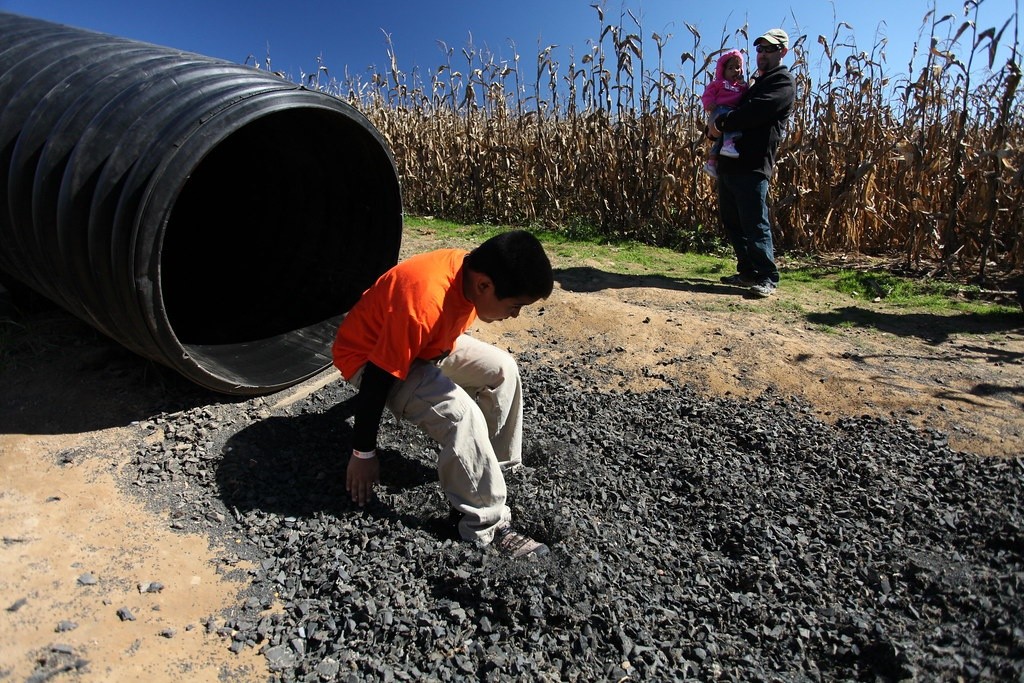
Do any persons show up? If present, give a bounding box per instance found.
[331,228,556,559]
[700,48,756,180]
[702,28,797,298]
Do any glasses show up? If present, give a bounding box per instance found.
[755,44,783,53]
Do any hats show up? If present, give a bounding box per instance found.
[752,29,789,48]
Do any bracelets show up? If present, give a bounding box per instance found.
[714,122,722,133]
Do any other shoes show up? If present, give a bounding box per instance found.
[750,278,776,297]
[701,160,718,179]
[720,138,739,157]
[720,274,755,287]
[493,526,550,561]
